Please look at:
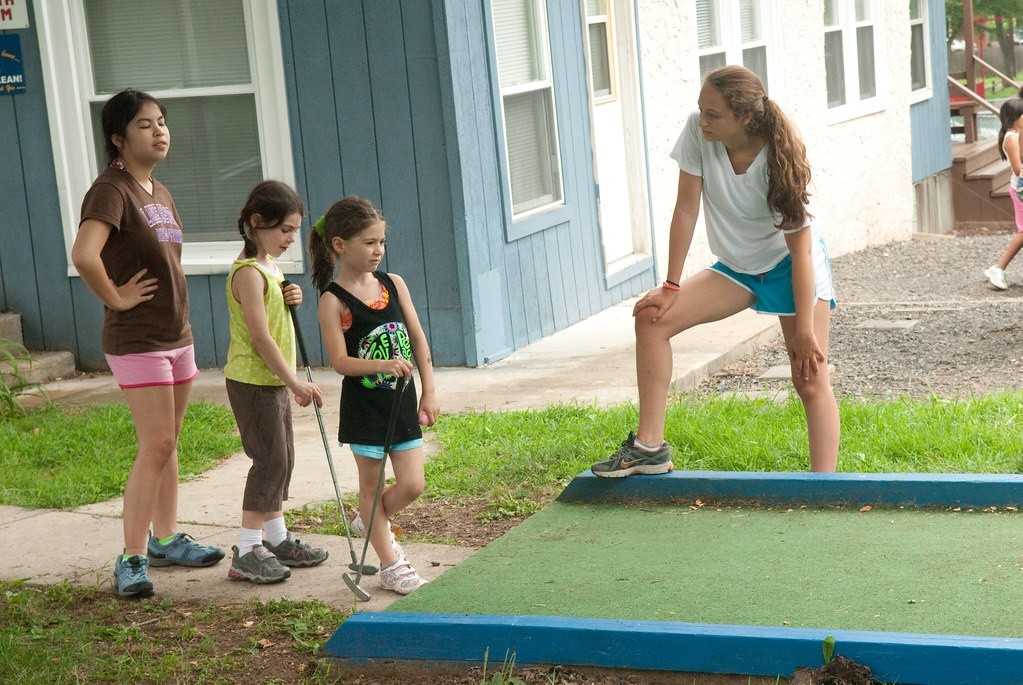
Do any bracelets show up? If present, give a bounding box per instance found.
[662,280,680,292]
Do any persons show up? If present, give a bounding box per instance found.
[71,92,225,597]
[591,65,841,479]
[310,199,440,594]
[986,98,1023,288]
[222,181,329,583]
[1016,125,1023,200]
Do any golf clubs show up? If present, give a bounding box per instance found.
[343,376,406,602]
[282,279,378,576]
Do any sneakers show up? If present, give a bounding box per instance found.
[115,553,152,597]
[349,513,404,558]
[590,436,673,478]
[263,534,329,566]
[147,533,224,566]
[986,265,1008,290]
[379,557,429,593]
[228,545,290,583]
